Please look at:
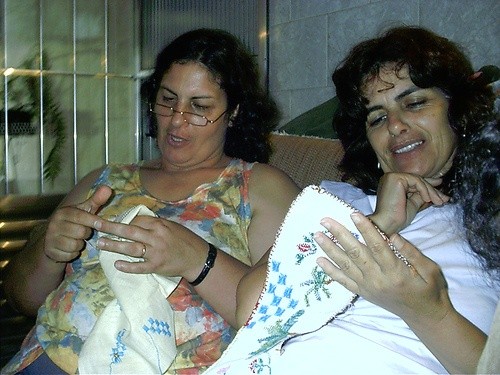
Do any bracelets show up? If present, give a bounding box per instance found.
[188,242,217,287]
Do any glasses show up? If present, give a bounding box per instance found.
[148,79,237,126]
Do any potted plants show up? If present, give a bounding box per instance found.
[1,43,69,189]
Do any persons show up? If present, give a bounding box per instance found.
[236,24,500,375]
[0,28,301,375]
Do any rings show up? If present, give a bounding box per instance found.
[140,243,146,258]
